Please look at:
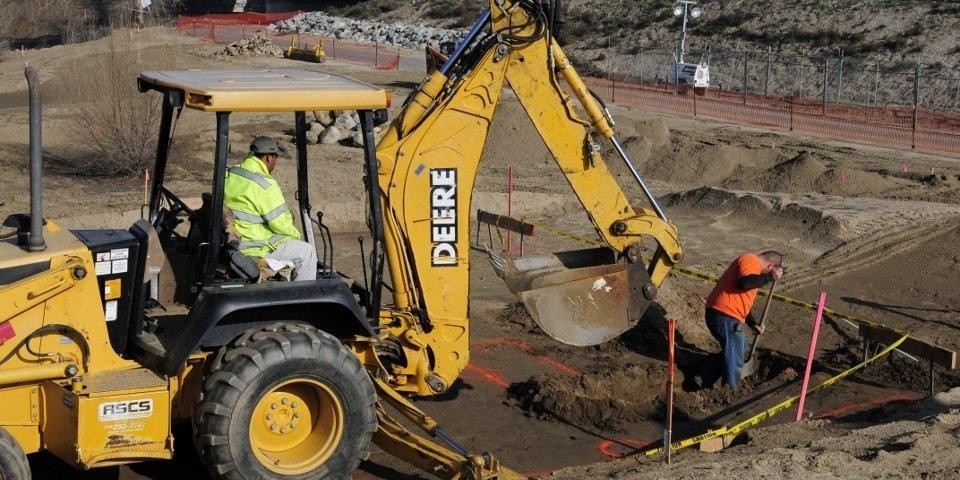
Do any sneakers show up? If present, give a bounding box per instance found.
[686,373,704,391]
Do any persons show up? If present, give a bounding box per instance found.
[704,249,784,389]
[223,134,319,283]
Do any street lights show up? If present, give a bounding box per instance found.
[672,0,702,62]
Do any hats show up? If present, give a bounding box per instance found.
[250,136,284,156]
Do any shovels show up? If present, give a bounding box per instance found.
[742,267,783,379]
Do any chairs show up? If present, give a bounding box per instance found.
[202,193,300,281]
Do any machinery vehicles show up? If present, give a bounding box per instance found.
[0,0,684,479]
[283,34,327,63]
